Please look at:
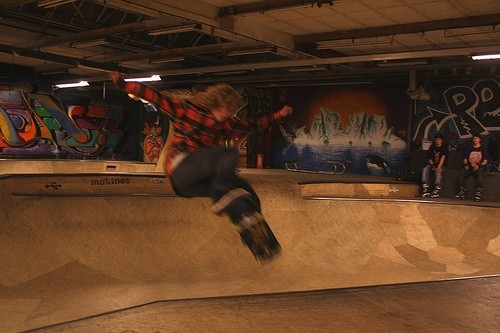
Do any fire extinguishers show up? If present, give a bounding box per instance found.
[257,154,263,169]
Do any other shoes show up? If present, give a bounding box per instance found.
[474,185,484,201]
[422,185,430,197]
[454,185,466,199]
[431,186,441,198]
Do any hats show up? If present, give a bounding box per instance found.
[434,133,442,138]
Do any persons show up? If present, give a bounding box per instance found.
[111,71,292,241]
[421,133,449,198]
[455,134,488,201]
